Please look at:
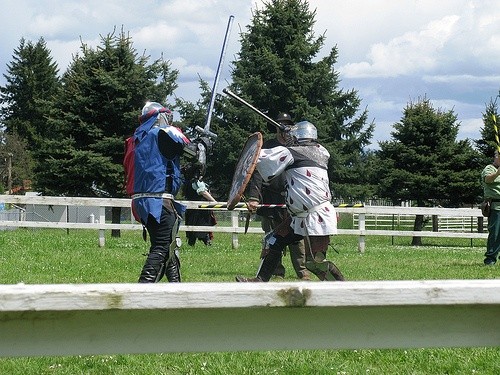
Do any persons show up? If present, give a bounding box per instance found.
[184,167,217,246]
[481,148,500,266]
[247,113,311,280]
[123,102,213,283]
[235,121,346,282]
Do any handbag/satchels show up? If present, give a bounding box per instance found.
[482,202,491,217]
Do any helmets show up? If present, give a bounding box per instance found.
[141,101,169,123]
[267,113,295,133]
[290,121,317,140]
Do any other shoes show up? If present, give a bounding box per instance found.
[236,276,263,282]
[484,258,496,266]
[274,275,283,280]
[300,275,310,280]
